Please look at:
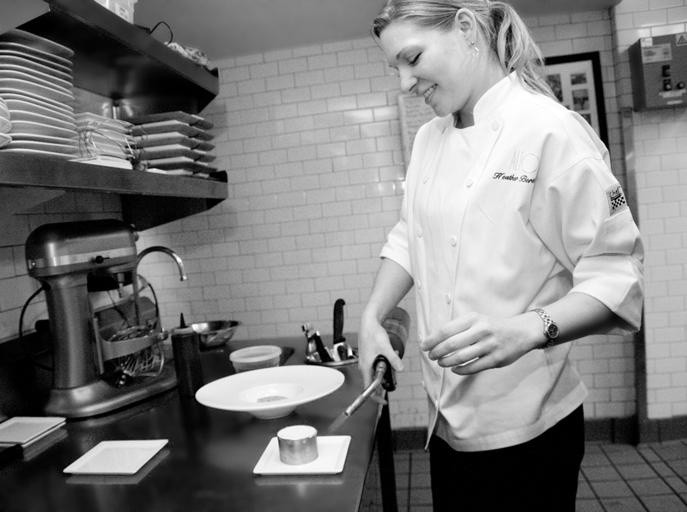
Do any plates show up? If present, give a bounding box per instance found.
[0,26,218,179]
[0,416,67,449]
[62,439,169,475]
[195,365,345,420]
[252,435,352,476]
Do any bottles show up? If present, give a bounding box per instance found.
[171,312,204,400]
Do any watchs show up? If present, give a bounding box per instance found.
[531,308,560,350]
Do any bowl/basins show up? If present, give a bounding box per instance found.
[229,345,282,373]
[190,320,240,350]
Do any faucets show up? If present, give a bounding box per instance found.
[132,248,186,333]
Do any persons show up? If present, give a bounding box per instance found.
[357,0,646,512]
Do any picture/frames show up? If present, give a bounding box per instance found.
[528,50,608,154]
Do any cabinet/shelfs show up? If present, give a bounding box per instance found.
[0,0,230,232]
